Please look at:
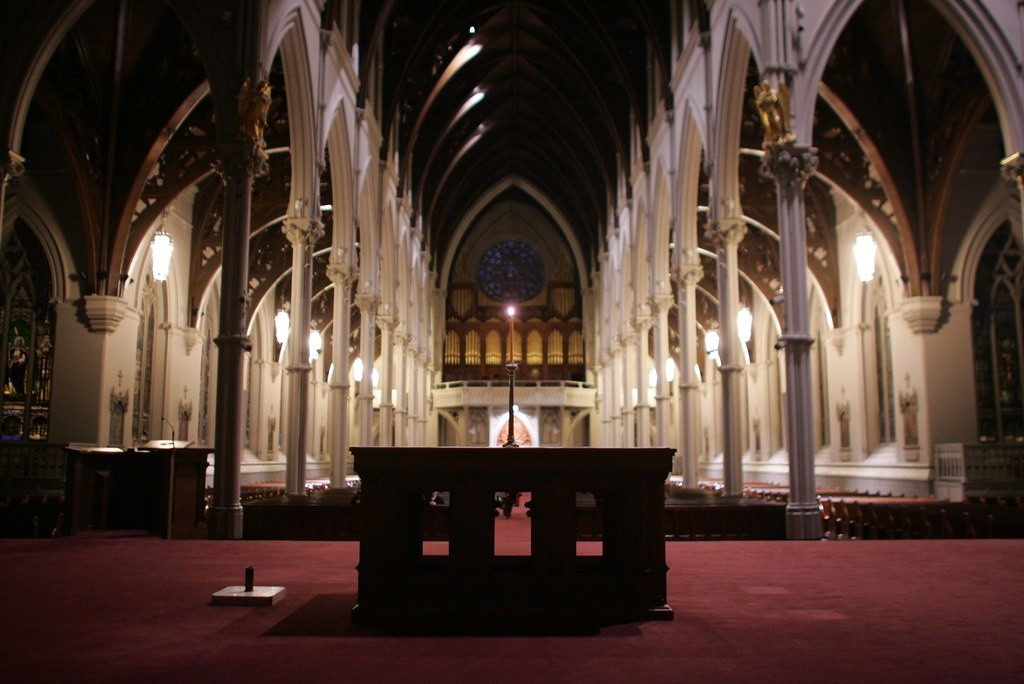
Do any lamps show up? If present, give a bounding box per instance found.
[149,210,174,282]
[274,280,291,344]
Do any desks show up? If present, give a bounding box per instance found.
[348,446,678,626]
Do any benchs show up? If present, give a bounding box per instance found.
[663,473,1024,541]
[204,474,499,541]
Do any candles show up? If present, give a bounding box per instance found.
[507,307,515,363]
